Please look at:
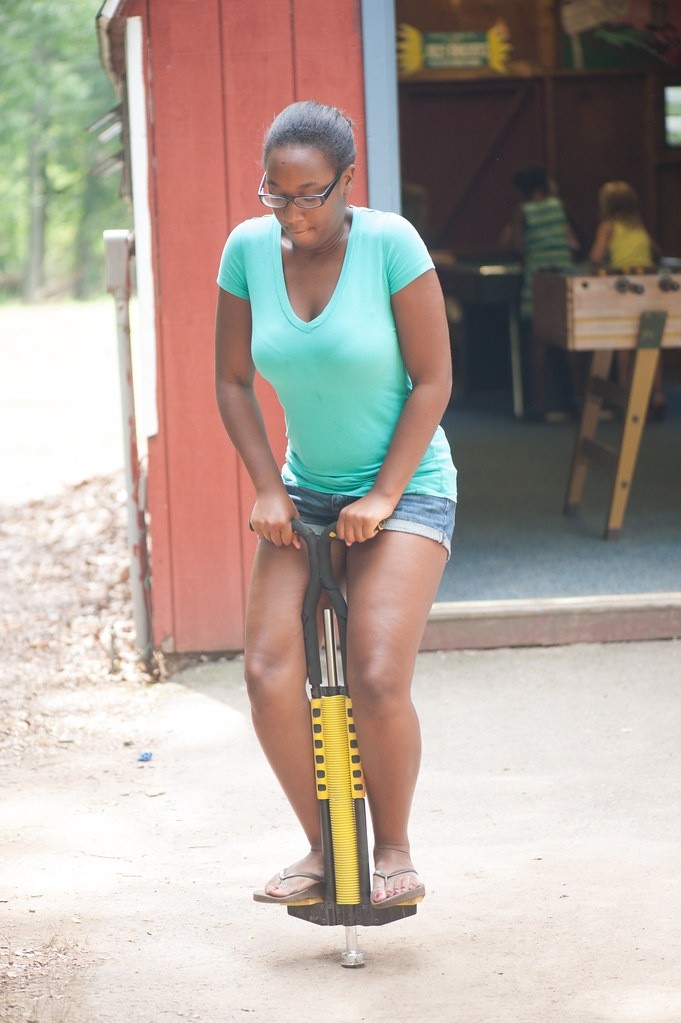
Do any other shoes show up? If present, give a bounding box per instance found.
[650,391,668,421]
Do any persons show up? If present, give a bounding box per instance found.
[510,167,583,415]
[215,100,459,909]
[590,180,667,419]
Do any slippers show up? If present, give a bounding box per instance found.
[370,868,425,910]
[253,867,325,903]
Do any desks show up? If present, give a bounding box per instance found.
[453,248,589,418]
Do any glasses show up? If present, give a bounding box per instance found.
[258,171,342,209]
